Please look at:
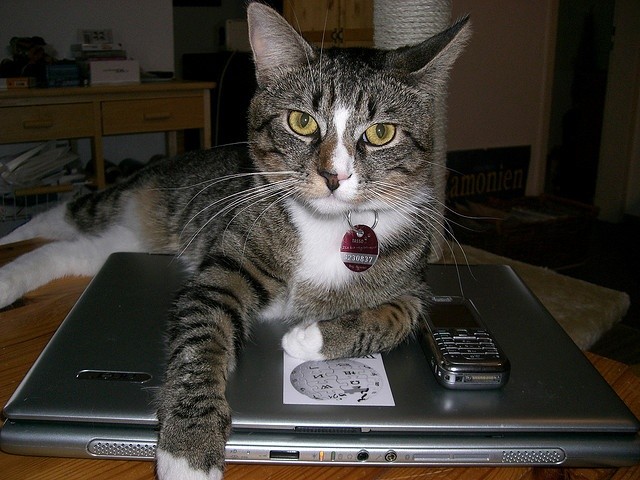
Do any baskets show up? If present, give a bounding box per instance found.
[444,193,600,263]
[0,177,80,222]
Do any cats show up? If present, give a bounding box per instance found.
[0,2,481,480]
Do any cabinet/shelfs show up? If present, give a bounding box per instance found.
[281,1,560,198]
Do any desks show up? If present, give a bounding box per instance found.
[0,74,218,223]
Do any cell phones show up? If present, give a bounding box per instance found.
[417,293,512,392]
